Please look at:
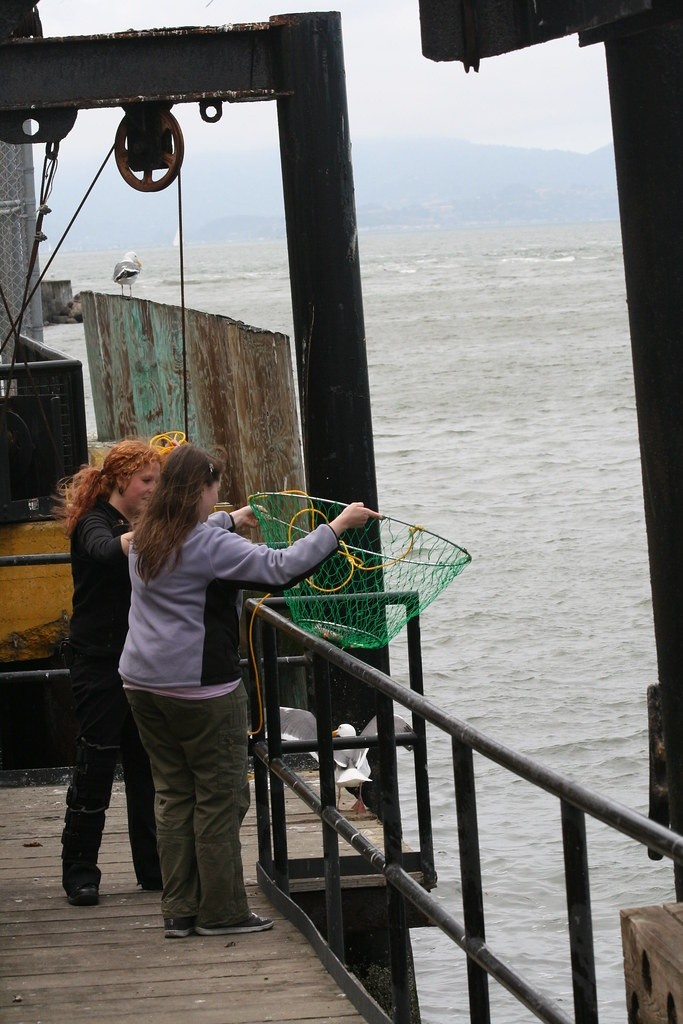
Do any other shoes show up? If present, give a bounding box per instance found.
[139,880,163,890]
[164,918,194,937]
[68,883,98,905]
[195,913,274,936]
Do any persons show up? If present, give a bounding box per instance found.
[116,441,384,939]
[51,444,163,906]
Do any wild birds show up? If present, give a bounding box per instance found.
[112,251,142,299]
